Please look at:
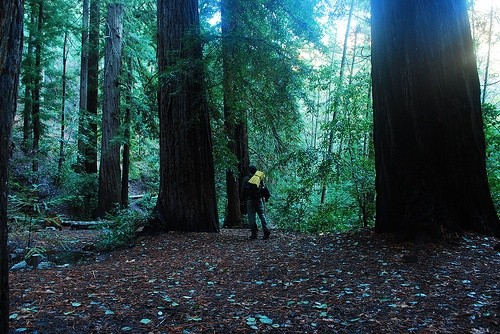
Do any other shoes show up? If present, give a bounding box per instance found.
[264,229,271,238]
[250,228,258,238]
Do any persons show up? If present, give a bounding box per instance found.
[242,165,273,241]
